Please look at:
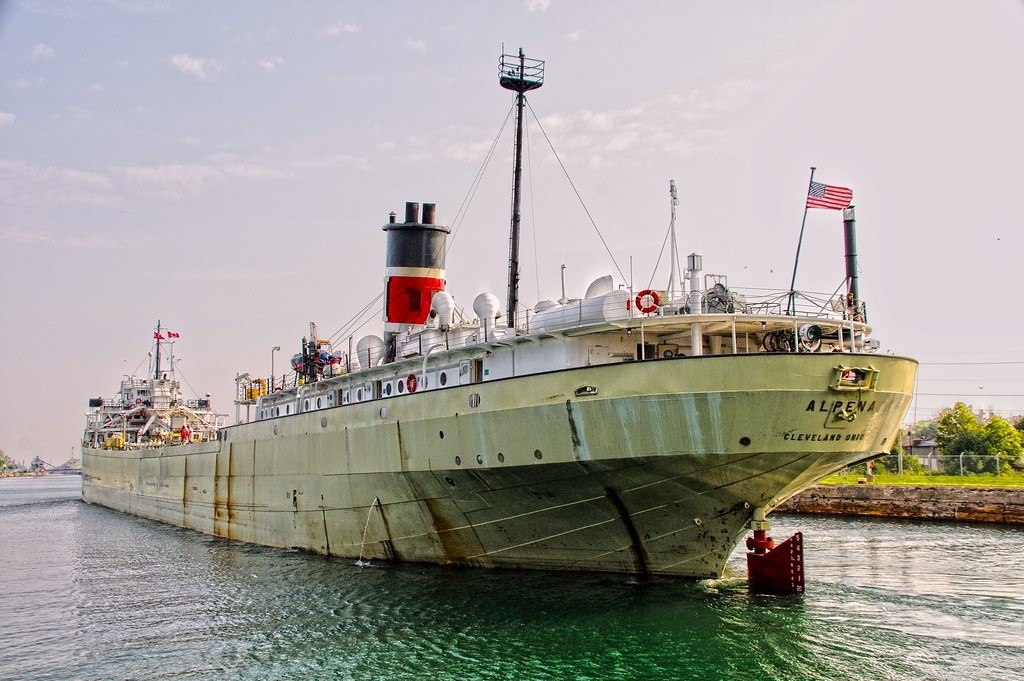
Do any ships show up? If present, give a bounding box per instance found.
[77,40,919,600]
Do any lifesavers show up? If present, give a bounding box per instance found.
[130,404,135,409]
[136,398,141,404]
[407,374,416,392]
[636,290,659,313]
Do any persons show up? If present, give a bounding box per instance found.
[142,399,151,410]
[169,400,177,408]
[385,333,393,364]
[388,332,396,364]
[83,424,219,452]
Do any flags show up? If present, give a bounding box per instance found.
[168,331,179,338]
[153,332,164,339]
[805,181,853,211]
